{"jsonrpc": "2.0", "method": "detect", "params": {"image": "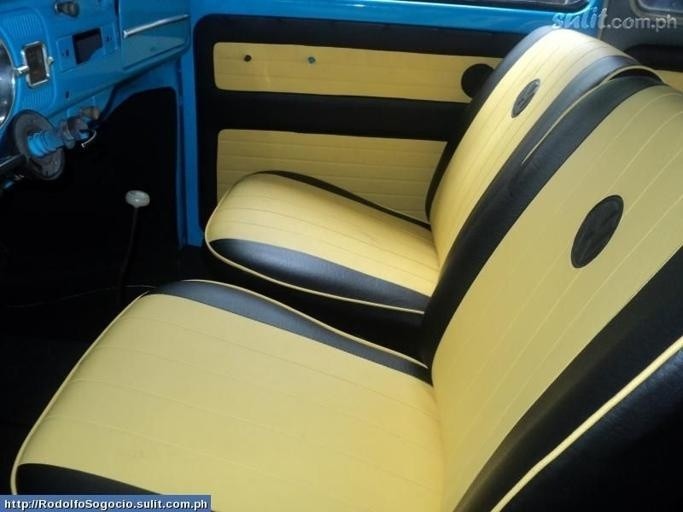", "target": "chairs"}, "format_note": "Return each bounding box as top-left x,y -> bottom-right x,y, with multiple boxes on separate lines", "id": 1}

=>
12,74 -> 680,508
202,22 -> 662,339
